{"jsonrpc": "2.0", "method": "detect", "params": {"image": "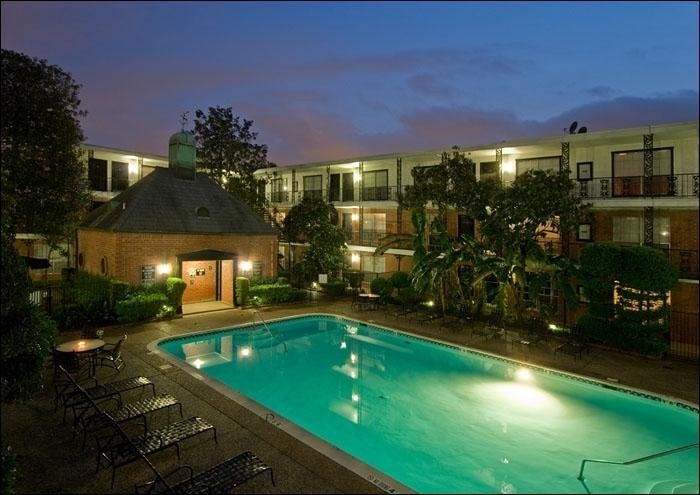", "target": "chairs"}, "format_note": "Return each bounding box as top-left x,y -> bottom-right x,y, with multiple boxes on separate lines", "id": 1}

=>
350,296 -> 589,360
53,335 -> 276,495
